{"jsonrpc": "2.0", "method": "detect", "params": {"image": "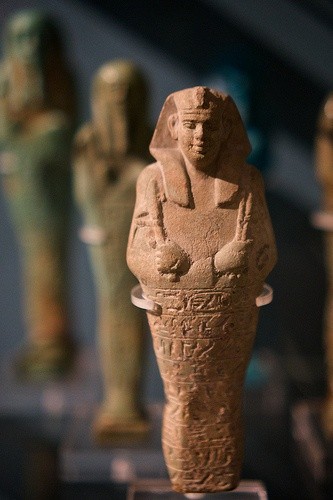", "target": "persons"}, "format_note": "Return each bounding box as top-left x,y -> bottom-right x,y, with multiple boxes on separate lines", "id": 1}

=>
0,10 -> 82,348
125,87 -> 279,492
71,58 -> 158,419
